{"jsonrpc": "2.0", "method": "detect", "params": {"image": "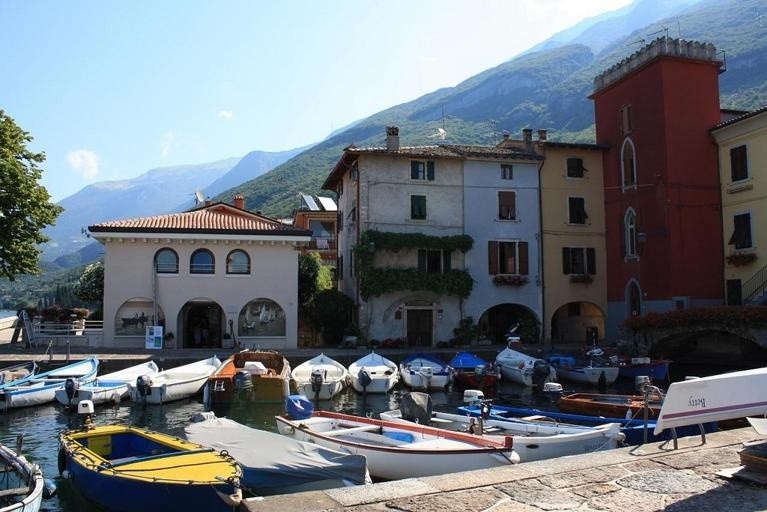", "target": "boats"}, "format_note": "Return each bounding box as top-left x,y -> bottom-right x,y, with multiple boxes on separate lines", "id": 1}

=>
202,347 -> 291,412
0,433 -> 44,512
544,350 -> 620,388
273,394 -> 521,483
182,410 -> 373,497
378,391 -> 626,464
0,355 -> 100,412
653,366 -> 767,436
455,389 -> 719,446
0,361 -> 40,401
495,345 -> 558,388
557,384 -> 667,419
348,349 -> 399,394
399,351 -> 454,392
57,399 -> 245,512
54,359 -> 160,414
289,351 -> 348,405
448,350 -> 502,388
584,348 -> 673,382
126,354 -> 222,410
